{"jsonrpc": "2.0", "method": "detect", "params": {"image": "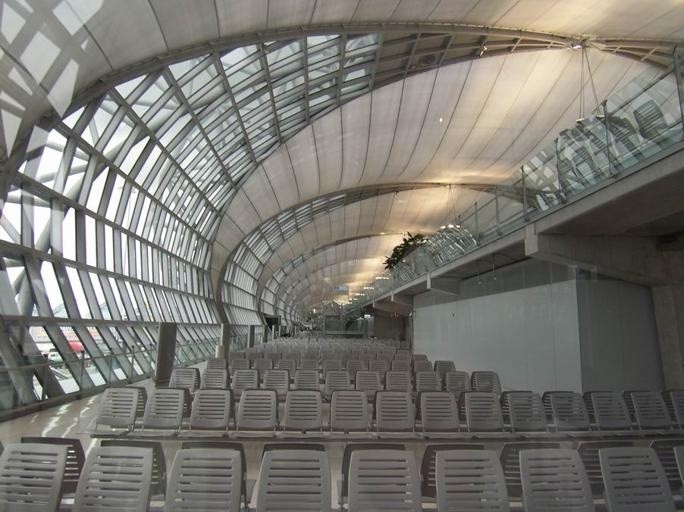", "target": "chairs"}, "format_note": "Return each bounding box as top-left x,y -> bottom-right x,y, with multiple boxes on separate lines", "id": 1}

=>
2,437 -> 684,512
154,337 -> 510,393
555,99 -> 683,199
90,388 -> 684,436
